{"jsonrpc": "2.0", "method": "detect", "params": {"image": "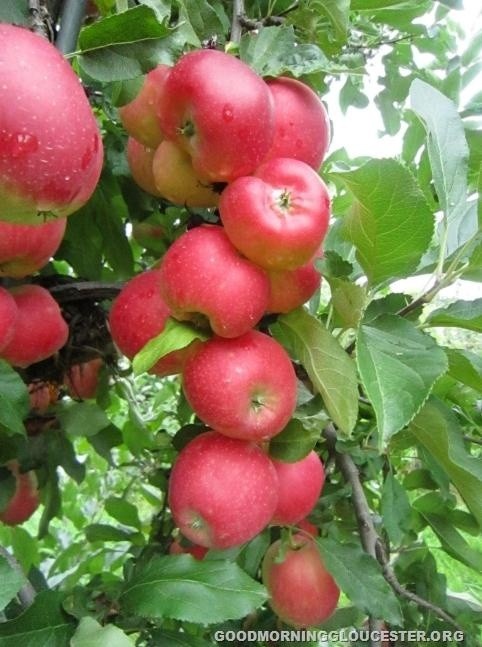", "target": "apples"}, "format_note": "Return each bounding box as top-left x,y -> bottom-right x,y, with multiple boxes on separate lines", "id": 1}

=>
0,0 -> 340,628
